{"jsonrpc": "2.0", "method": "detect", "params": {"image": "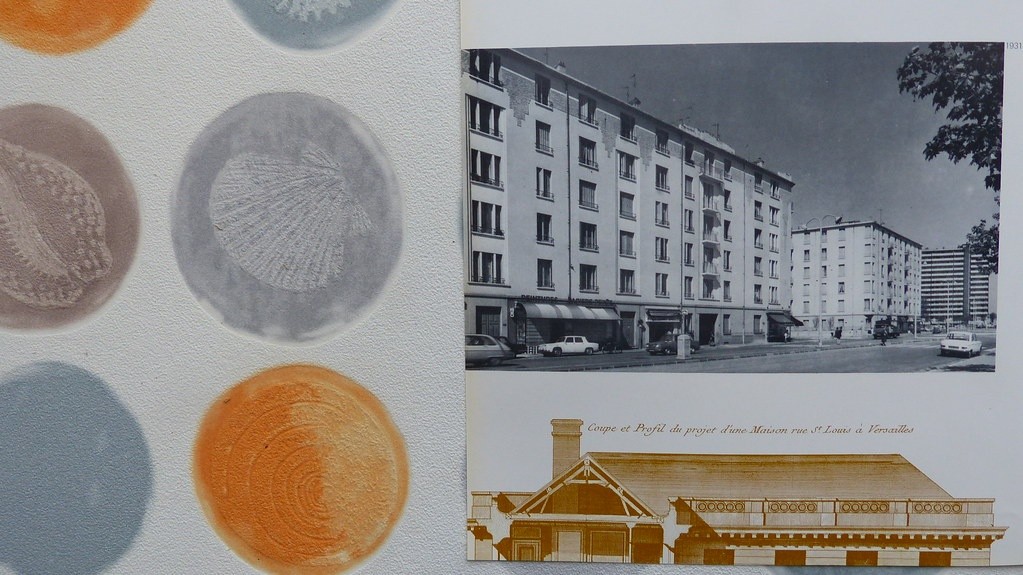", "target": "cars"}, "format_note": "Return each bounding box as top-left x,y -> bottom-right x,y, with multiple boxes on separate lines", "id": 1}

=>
465,333 -> 514,367
645,333 -> 701,355
873,323 -> 901,340
539,336 -> 600,357
940,331 -> 983,358
488,335 -> 526,358
